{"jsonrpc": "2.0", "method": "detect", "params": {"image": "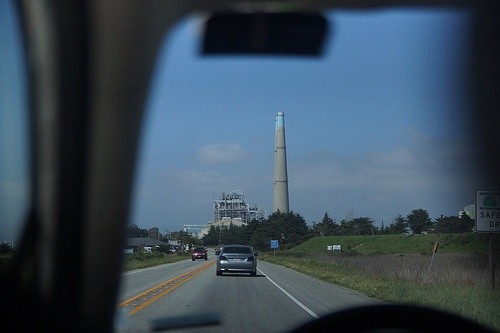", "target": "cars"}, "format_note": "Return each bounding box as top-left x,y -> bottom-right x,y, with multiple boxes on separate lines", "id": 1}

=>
191,246 -> 209,261
213,245 -> 259,277
214,248 -> 220,255
